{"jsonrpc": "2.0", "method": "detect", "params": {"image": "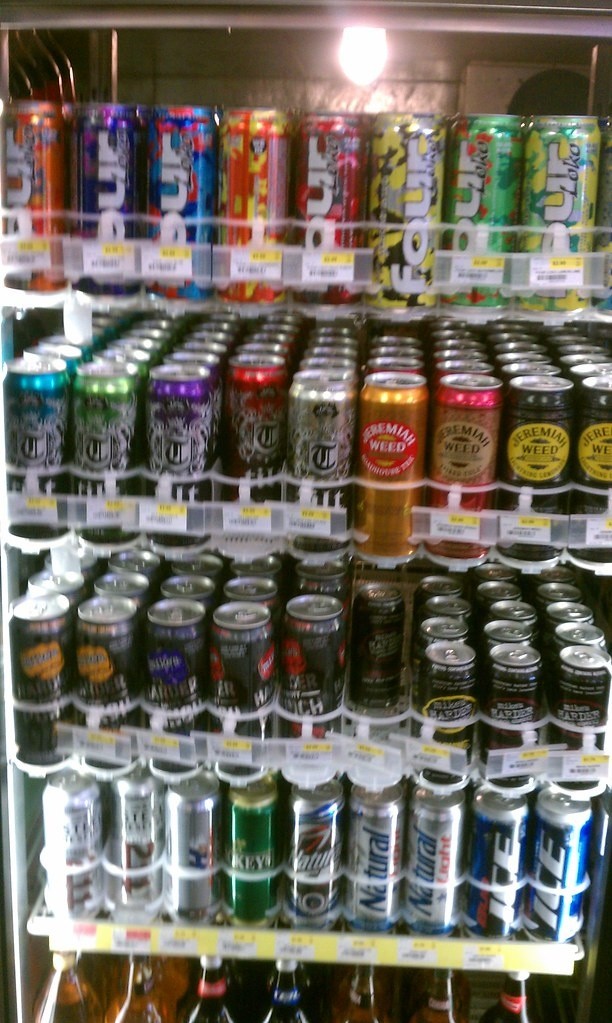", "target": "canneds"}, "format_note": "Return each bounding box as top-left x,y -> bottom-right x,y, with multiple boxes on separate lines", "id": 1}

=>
2,97 -> 611,320
0,312 -> 612,942
